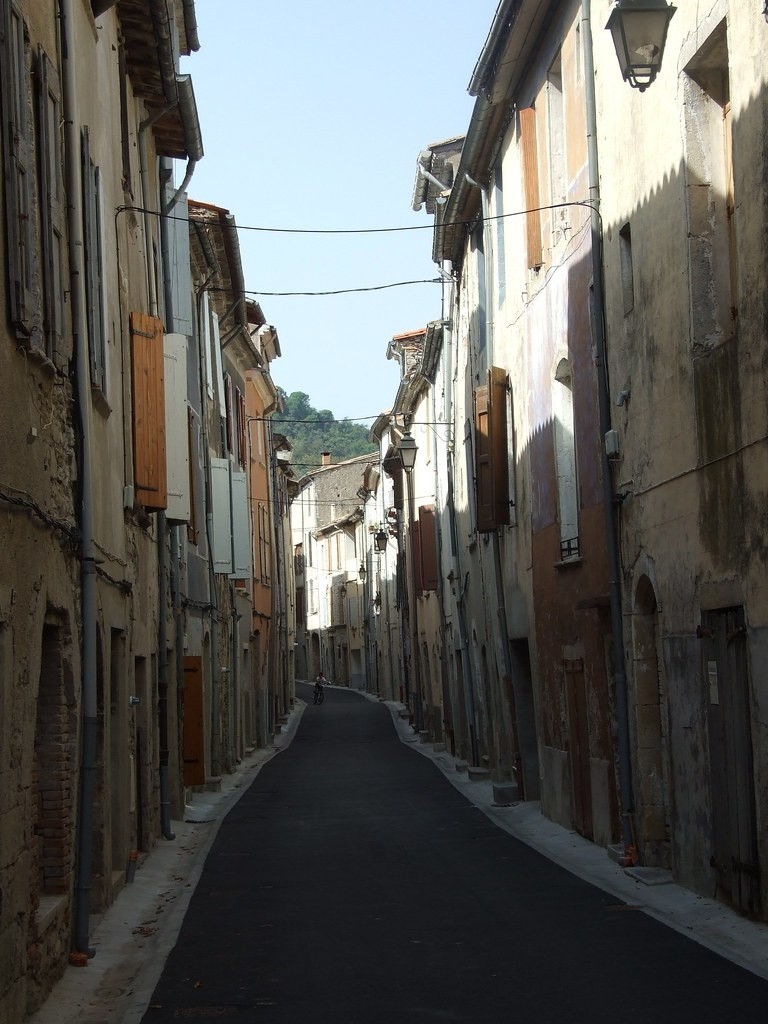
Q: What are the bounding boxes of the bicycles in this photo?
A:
[312,680,328,705]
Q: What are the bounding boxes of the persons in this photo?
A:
[312,672,326,703]
